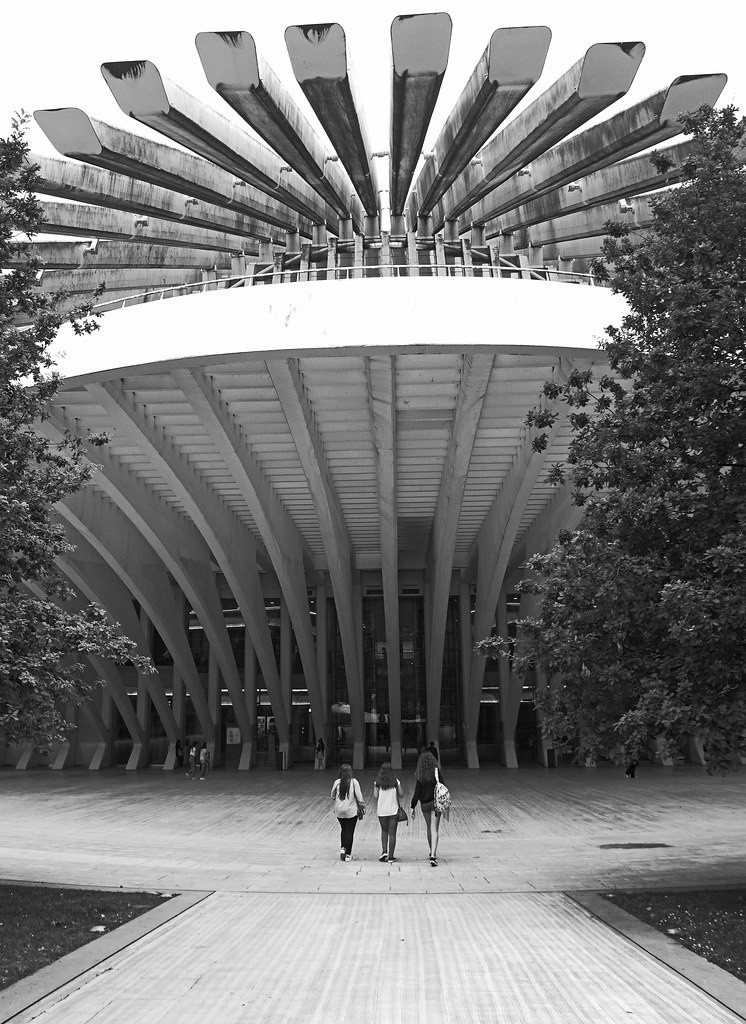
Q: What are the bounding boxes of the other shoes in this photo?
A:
[345,855,355,861]
[388,857,397,863]
[378,853,387,861]
[340,848,345,860]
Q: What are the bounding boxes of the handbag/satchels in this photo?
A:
[357,805,363,820]
[398,807,409,826]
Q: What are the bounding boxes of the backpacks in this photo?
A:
[433,766,452,812]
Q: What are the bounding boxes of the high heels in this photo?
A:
[429,857,438,867]
[429,853,437,862]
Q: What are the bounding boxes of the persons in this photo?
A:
[186,741,200,779]
[427,742,438,761]
[410,751,446,867]
[175,739,184,770]
[624,759,639,779]
[378,711,385,723]
[183,738,191,775]
[199,742,210,780]
[315,738,325,771]
[331,764,366,862]
[373,762,404,863]
